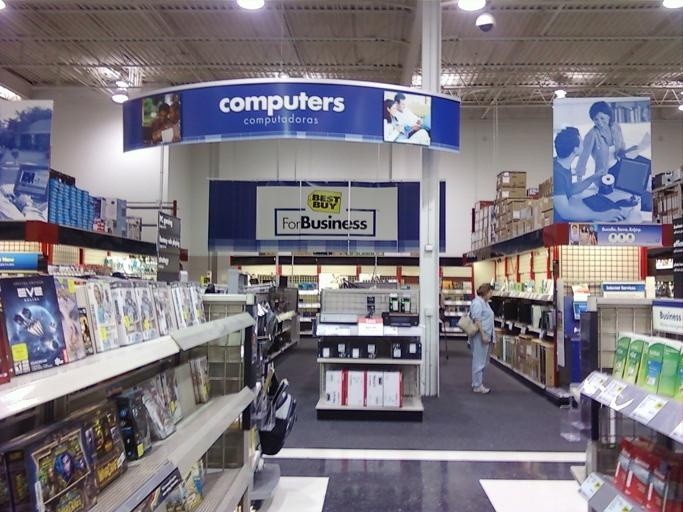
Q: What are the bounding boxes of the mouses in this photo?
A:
[611,216,626,222]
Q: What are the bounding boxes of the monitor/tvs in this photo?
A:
[615,158,651,207]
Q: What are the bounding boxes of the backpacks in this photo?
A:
[258,361,297,455]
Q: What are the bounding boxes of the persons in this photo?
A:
[150,102,181,145]
[470,283,495,394]
[384,93,431,144]
[553,101,626,222]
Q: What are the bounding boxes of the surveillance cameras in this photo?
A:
[475,13,495,32]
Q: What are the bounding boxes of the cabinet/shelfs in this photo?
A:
[570,296,683,512]
[469,222,664,406]
[315,280,427,420]
[2,218,324,512]
[438,256,473,338]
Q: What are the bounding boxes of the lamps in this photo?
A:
[112,81,129,104]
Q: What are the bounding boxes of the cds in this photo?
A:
[601,174,615,186]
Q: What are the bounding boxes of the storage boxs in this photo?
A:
[470,171,553,251]
[653,167,683,225]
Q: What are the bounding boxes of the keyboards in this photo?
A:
[582,193,621,213]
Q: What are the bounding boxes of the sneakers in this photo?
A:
[472,384,490,393]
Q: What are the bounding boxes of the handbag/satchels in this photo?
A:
[457,314,478,337]
